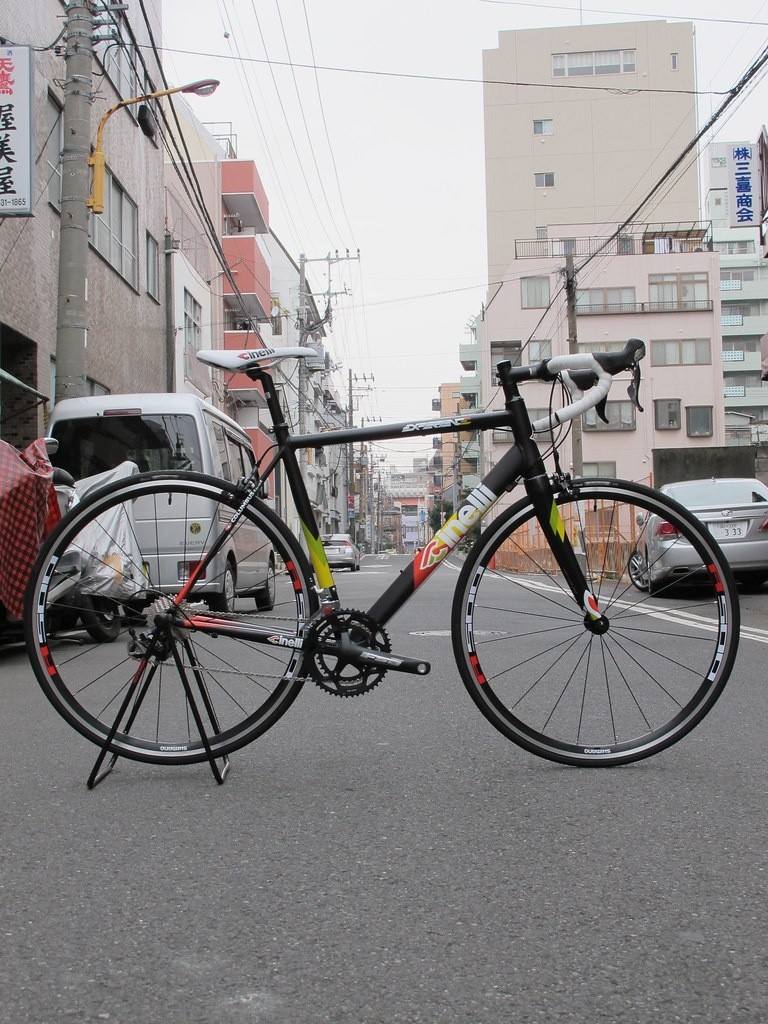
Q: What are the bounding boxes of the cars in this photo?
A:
[319,533,360,571]
[627,477,768,595]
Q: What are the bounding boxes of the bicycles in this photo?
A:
[20,343,741,789]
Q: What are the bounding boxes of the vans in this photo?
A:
[45,393,276,616]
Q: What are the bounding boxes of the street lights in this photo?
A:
[54,1,219,396]
